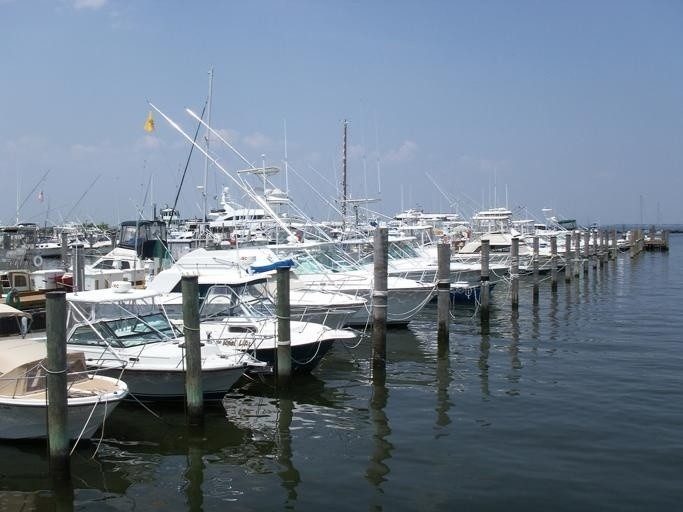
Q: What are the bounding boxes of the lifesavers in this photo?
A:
[437,230,448,241]
[6,288,21,308]
